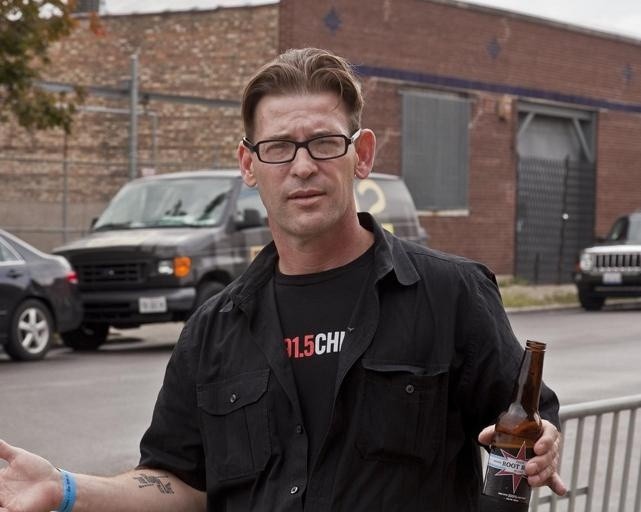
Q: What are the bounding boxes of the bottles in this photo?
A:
[478,339,547,512]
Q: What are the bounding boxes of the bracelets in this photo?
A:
[55,468,76,511]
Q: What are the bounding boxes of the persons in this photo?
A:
[0,47,567,512]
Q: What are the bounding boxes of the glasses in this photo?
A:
[241,128,369,164]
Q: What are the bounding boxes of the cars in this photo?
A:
[0,231,83,363]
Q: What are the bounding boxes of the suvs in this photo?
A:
[573,211,640,311]
[51,167,427,350]
